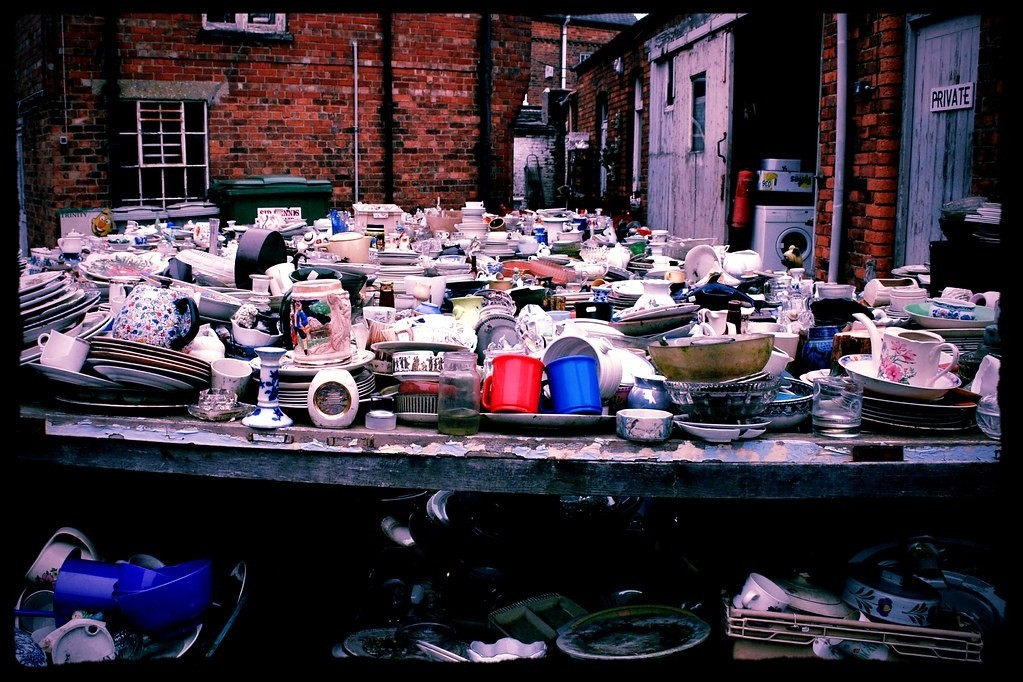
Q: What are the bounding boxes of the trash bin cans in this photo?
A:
[210,176,334,226]
[112,203,219,231]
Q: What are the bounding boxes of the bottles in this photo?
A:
[633,280,676,311]
[439,353,480,435]
[290,280,353,366]
[801,325,841,372]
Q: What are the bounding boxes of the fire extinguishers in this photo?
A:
[732,168,756,227]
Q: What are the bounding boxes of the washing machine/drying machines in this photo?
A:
[753,206,814,275]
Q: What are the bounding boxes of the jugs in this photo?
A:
[112,278,200,350]
[314,233,373,264]
[852,313,961,387]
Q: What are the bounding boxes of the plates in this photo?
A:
[334,590,710,661]
[18,206,1002,440]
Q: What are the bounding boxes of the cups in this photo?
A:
[539,355,603,415]
[738,572,789,612]
[481,354,545,415]
[37,327,90,373]
[811,375,864,437]
[210,358,254,401]
[19,527,117,665]
[830,609,896,660]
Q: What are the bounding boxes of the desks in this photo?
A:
[929,240,1003,299]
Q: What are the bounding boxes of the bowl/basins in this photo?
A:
[394,350,446,372]
[391,392,440,412]
[230,317,285,348]
[112,558,213,625]
[616,408,674,441]
[846,565,941,626]
[662,374,792,422]
[647,332,776,383]
